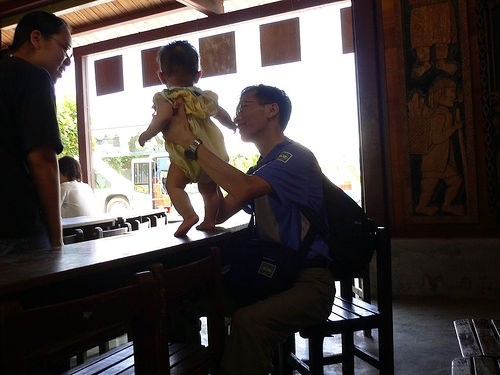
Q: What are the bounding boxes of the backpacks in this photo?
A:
[289,175,374,281]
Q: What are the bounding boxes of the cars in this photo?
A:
[73,154,149,220]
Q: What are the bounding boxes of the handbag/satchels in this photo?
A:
[218,228,302,306]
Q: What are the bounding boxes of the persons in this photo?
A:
[0,10,72,248]
[138,39,240,238]
[152,83,336,375]
[58,155,99,218]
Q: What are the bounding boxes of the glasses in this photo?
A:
[42,32,72,59]
[236,101,272,115]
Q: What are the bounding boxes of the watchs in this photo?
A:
[184,137,204,162]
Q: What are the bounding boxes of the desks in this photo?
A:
[62,209,167,244]
[0,222,249,375]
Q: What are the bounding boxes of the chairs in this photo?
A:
[449,319,500,375]
[0,213,392,375]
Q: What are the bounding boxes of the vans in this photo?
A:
[130,150,176,215]
